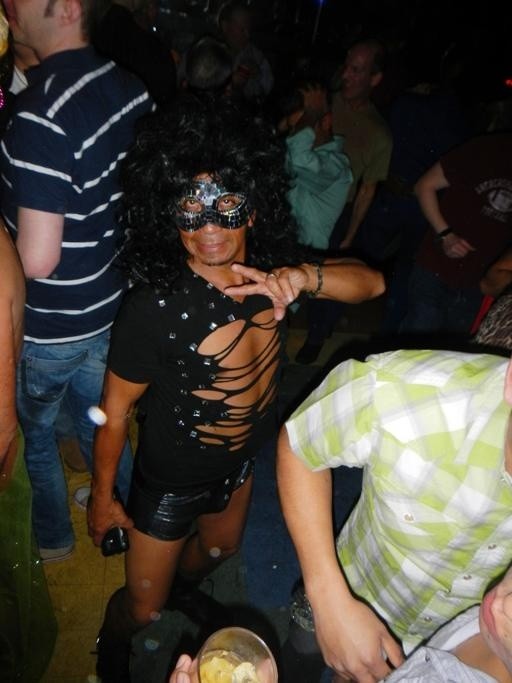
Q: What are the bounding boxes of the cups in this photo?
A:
[188,624,278,682]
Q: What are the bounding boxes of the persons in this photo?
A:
[1,217,60,680]
[163,563,509,680]
[277,347,511,681]
[83,103,390,682]
[1,1,511,346]
[0,0,159,564]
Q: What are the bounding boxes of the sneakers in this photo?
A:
[36,546,75,562]
[73,487,92,511]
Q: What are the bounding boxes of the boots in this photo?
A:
[97,585,161,682]
